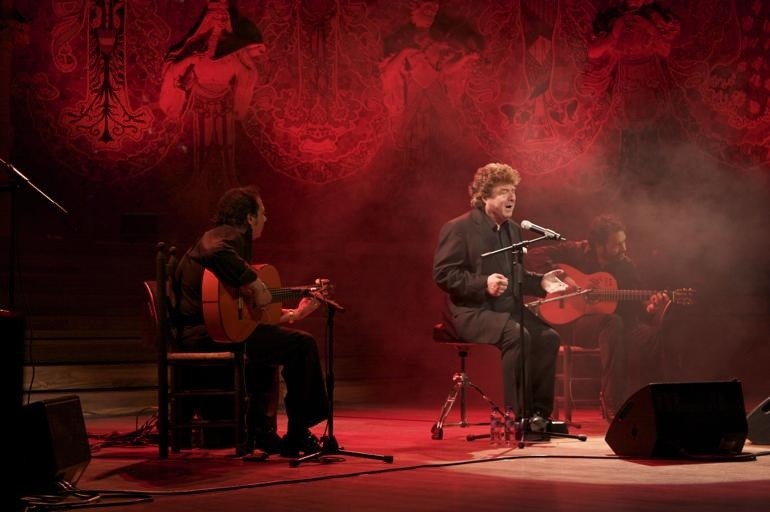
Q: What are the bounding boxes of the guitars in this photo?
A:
[201,263,335,345]
[538,263,697,326]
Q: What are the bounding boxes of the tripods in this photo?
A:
[291,320,394,464]
[468,281,587,445]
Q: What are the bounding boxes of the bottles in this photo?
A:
[191,414,205,450]
[489,407,502,443]
[505,406,517,444]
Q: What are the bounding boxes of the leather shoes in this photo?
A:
[515,417,555,442]
[245,430,325,457]
[597,388,619,425]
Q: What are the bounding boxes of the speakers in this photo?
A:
[747,395,770,444]
[605,380,749,457]
[27,395,91,473]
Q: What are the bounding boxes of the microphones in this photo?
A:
[521,220,564,241]
[303,288,345,314]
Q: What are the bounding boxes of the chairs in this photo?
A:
[137,274,279,459]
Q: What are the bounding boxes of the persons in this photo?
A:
[526,214,670,421]
[171,187,344,458]
[431,162,570,441]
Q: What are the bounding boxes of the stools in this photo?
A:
[423,320,510,442]
[549,342,603,424]
